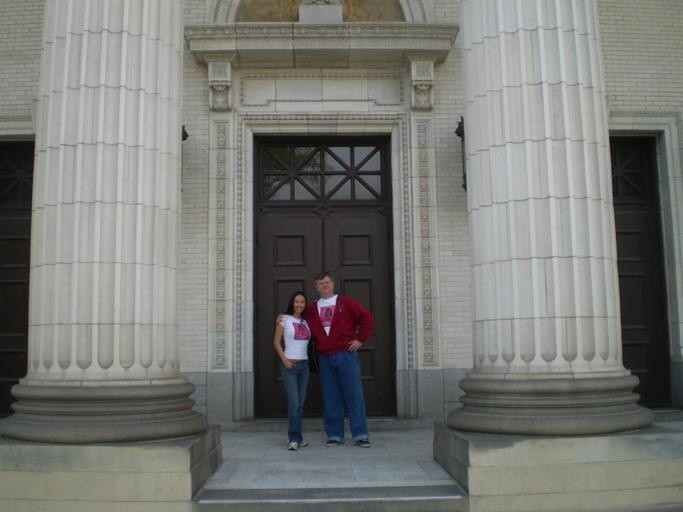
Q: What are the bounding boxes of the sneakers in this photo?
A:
[326,439,342,447]
[355,439,371,447]
[287,440,308,450]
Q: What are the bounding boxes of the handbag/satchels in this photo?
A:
[307,340,319,373]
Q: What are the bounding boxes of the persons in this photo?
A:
[275,270,374,448]
[273,291,312,451]
